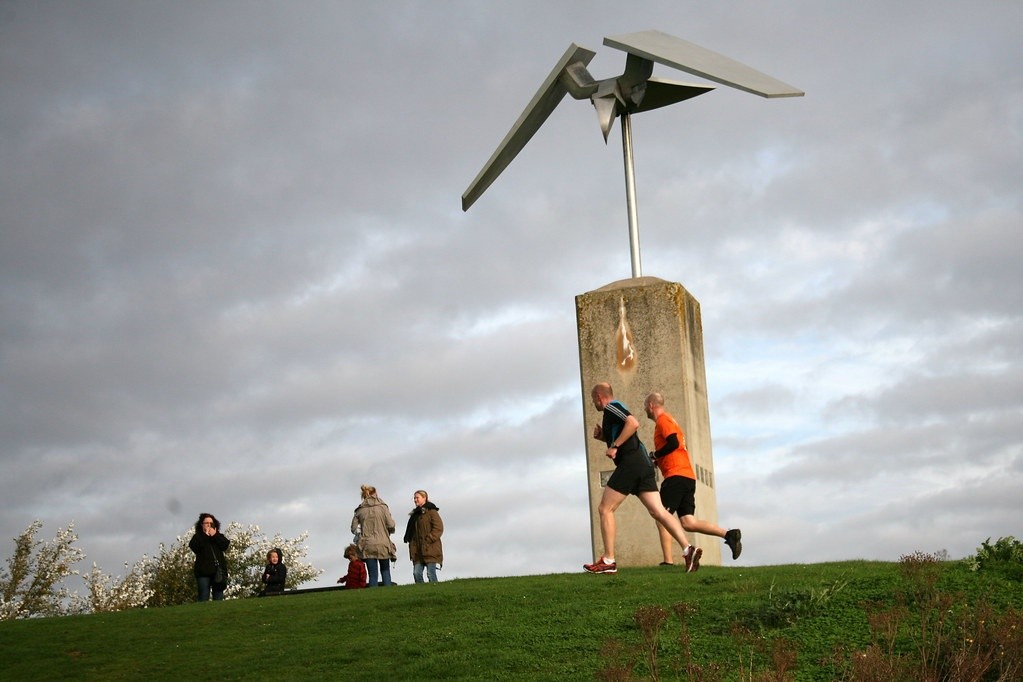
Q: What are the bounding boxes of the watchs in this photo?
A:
[650,452,656,458]
[611,442,618,448]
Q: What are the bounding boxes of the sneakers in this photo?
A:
[683,546,702,572]
[583,557,618,574]
[725,529,742,560]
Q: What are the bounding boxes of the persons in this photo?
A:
[337,544,367,591]
[583,383,702,576]
[644,392,742,565]
[258,548,286,595]
[404,490,444,584]
[188,513,229,602]
[351,485,395,586]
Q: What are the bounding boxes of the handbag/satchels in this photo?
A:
[213,567,227,590]
